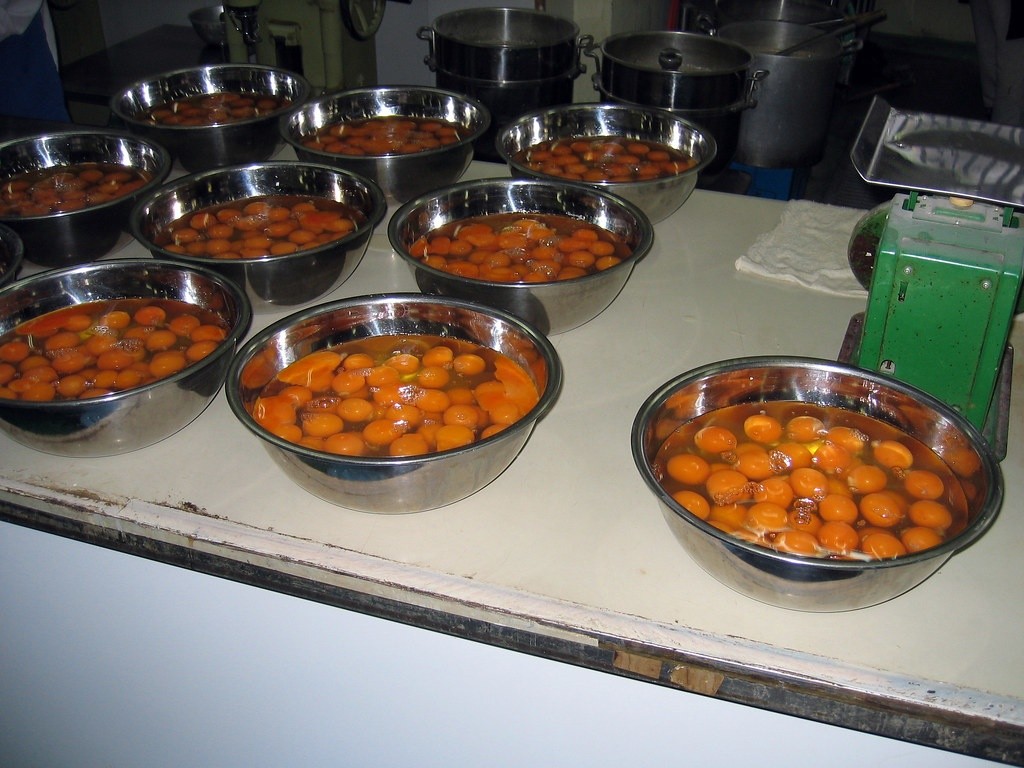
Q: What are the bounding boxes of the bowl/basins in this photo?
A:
[387,176,654,337]
[187,5,228,49]
[110,63,310,174]
[225,292,564,514]
[0,225,24,290]
[495,104,717,226]
[631,355,1004,612]
[131,160,387,317]
[0,129,172,267]
[0,258,253,457]
[281,86,491,204]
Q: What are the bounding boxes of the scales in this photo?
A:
[836,95,1024,463]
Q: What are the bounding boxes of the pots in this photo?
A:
[680,0,863,87]
[417,6,593,164]
[583,30,769,174]
[694,13,863,167]
